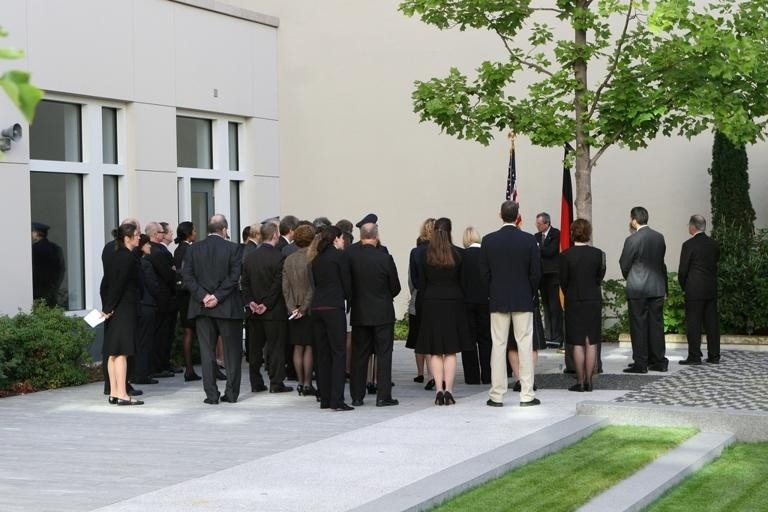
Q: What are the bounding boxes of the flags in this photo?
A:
[505,138,523,231]
[559,144,574,314]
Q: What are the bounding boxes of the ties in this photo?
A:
[541,233,546,247]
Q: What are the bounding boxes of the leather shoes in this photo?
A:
[442,380,446,390]
[172,366,184,373]
[127,389,142,396]
[586,383,594,392]
[221,394,237,403]
[116,395,144,405]
[487,399,505,407]
[563,367,578,375]
[414,375,424,384]
[108,395,117,403]
[707,357,720,365]
[331,401,355,411]
[532,384,536,390]
[425,379,436,391]
[678,357,703,366]
[321,401,331,409]
[627,363,637,367]
[377,397,399,407]
[568,383,585,392]
[622,364,648,374]
[352,399,364,407]
[215,368,226,380]
[204,397,219,405]
[649,359,670,373]
[251,384,268,393]
[129,376,159,385]
[184,372,202,381]
[150,369,175,378]
[270,385,293,393]
[513,381,522,392]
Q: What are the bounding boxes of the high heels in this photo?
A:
[296,384,303,396]
[444,391,456,406]
[304,385,318,396]
[373,383,377,395]
[434,391,444,406]
[314,391,323,402]
[366,382,372,394]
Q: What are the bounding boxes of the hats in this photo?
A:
[30,220,51,231]
[259,215,280,226]
[355,213,378,227]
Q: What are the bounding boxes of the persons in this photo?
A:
[160,222,184,374]
[275,215,299,381]
[627,221,637,367]
[130,234,159,386]
[182,214,245,404]
[622,206,669,373]
[405,236,426,382]
[216,335,225,370]
[559,219,605,393]
[344,223,398,407]
[348,214,395,394]
[480,201,541,407]
[241,220,293,393]
[243,226,250,243]
[242,223,264,364]
[282,221,316,256]
[414,216,464,407]
[410,218,447,390]
[678,215,721,365]
[145,221,175,377]
[282,224,317,396]
[336,219,353,384]
[309,226,355,410]
[313,218,328,233]
[263,218,280,237]
[174,221,227,382]
[507,285,543,392]
[461,226,492,385]
[533,212,563,349]
[31,222,66,310]
[307,230,320,401]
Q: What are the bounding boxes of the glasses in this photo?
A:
[535,222,546,227]
[157,231,166,234]
